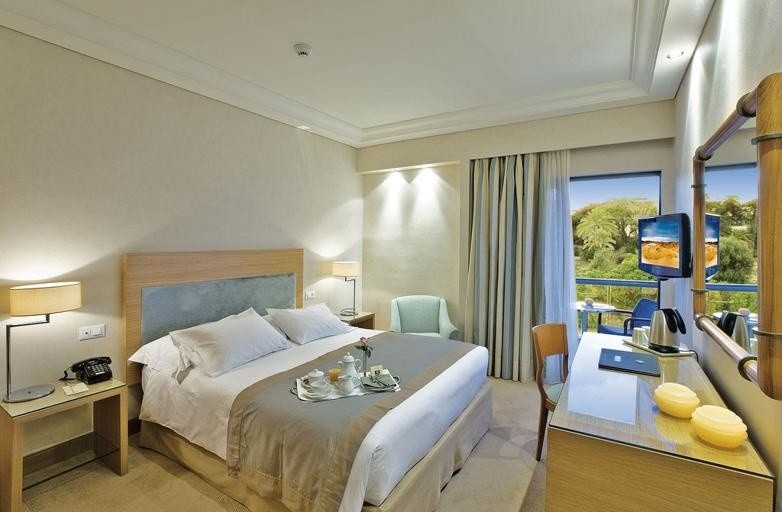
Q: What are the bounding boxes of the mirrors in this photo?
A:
[690,73,782,398]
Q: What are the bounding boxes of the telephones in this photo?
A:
[71,357,112,384]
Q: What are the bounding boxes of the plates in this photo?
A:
[303,390,328,400]
[302,374,330,388]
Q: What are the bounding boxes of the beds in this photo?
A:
[116,248,492,512]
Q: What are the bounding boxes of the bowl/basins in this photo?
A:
[585,297,594,305]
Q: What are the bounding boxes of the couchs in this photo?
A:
[387,293,458,343]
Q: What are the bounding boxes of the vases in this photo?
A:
[362,351,367,378]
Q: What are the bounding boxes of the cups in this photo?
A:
[328,368,341,383]
[632,327,650,347]
[307,368,325,383]
[750,338,759,354]
[309,381,328,395]
[641,325,650,337]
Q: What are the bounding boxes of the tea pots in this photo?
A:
[337,375,362,396]
[336,352,362,387]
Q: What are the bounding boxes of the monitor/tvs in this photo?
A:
[704,213,721,282]
[637,212,691,278]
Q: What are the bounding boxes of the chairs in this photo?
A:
[529,321,574,462]
[596,296,657,337]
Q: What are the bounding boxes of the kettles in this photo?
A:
[649,307,688,356]
[716,312,750,353]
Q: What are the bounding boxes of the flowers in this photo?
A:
[354,337,374,378]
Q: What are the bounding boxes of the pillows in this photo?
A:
[166,308,293,376]
[126,328,194,384]
[264,301,357,345]
[260,312,289,341]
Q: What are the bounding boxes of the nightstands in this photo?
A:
[339,312,378,332]
[0,378,132,512]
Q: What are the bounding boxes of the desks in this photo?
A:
[539,328,782,512]
[571,298,614,334]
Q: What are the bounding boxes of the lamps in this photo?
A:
[0,281,82,404]
[325,260,366,316]
[292,42,312,60]
